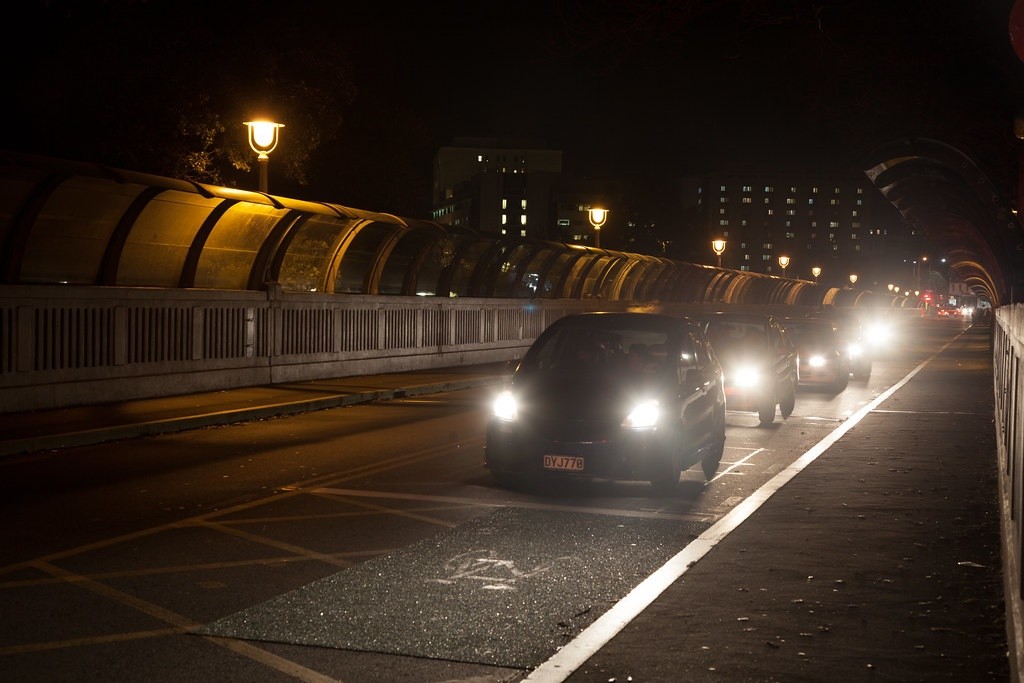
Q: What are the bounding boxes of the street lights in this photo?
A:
[851,274,920,299]
[242,114,285,193]
[587,203,610,249]
[779,254,790,278]
[712,237,727,267]
[812,265,822,283]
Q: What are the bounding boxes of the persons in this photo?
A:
[573,340,596,371]
[627,344,656,375]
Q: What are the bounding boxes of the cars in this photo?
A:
[778,316,847,390]
[695,313,799,423]
[482,310,726,492]
[806,303,985,382]
